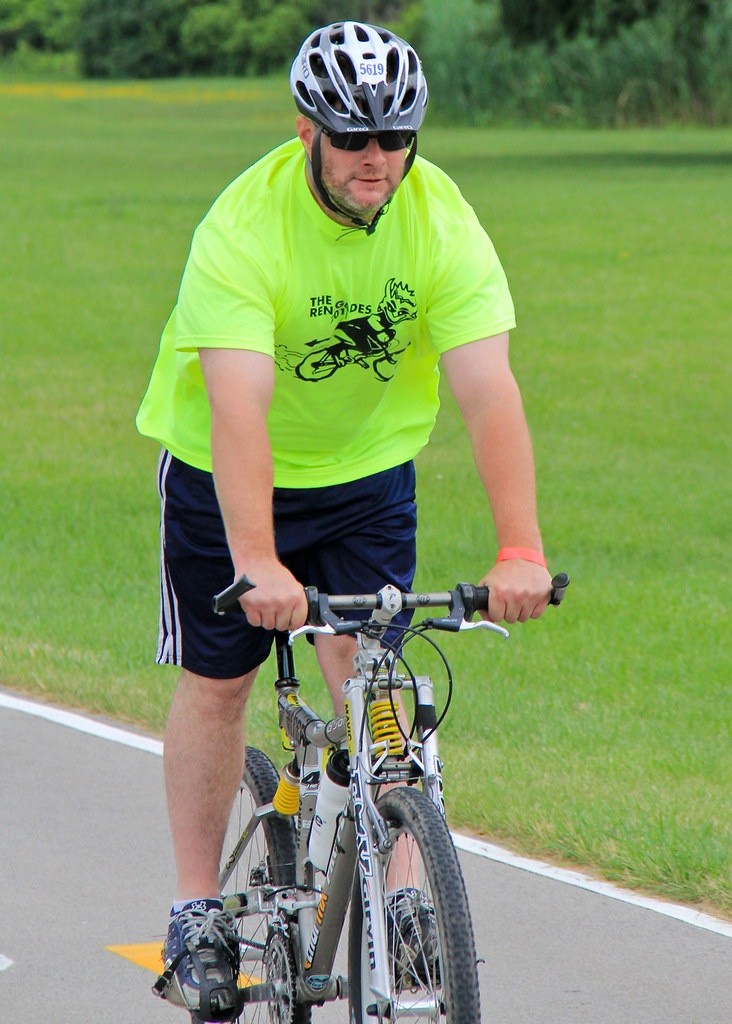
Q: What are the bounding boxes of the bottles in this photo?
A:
[308,749,353,871]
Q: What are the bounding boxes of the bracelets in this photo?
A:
[497,547,546,568]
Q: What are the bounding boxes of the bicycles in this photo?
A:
[189,567,571,1024]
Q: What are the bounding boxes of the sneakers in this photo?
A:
[161,899,240,1011]
[386,887,442,992]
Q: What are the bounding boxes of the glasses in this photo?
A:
[309,119,413,151]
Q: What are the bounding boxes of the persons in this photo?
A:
[134,23,551,1019]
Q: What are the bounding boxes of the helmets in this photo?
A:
[290,21,430,133]
